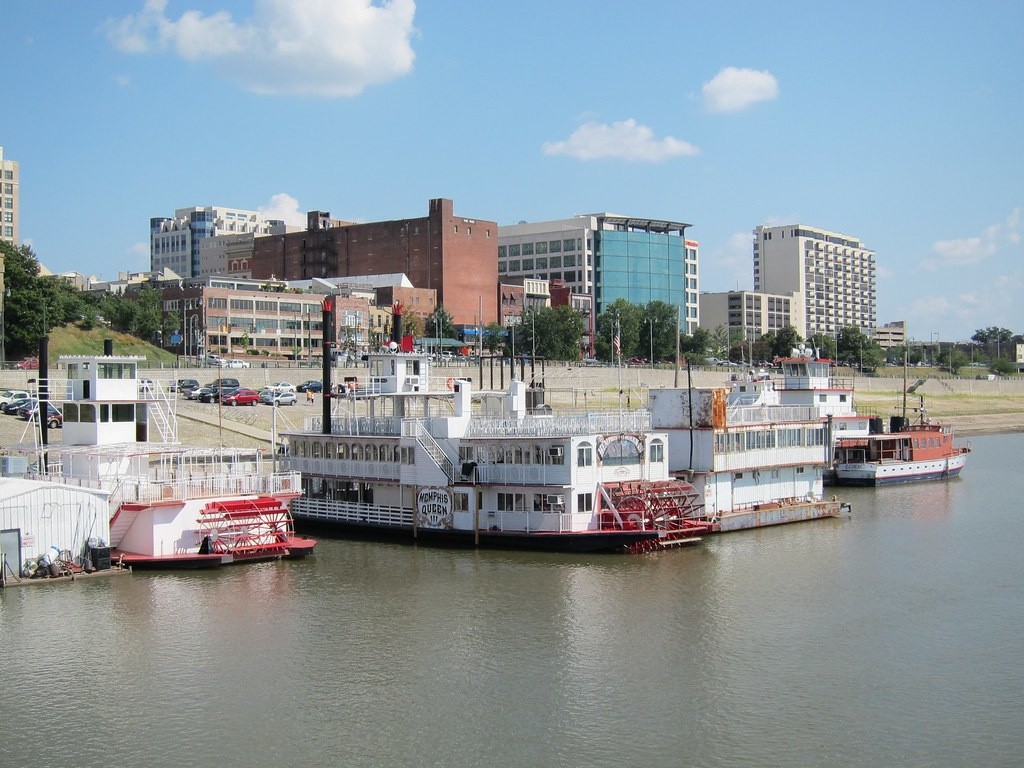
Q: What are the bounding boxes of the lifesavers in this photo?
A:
[447,378,455,389]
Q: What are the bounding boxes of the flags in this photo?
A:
[614,328,622,357]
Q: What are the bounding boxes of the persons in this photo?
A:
[305,381,336,406]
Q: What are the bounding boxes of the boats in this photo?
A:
[1,352,971,590]
